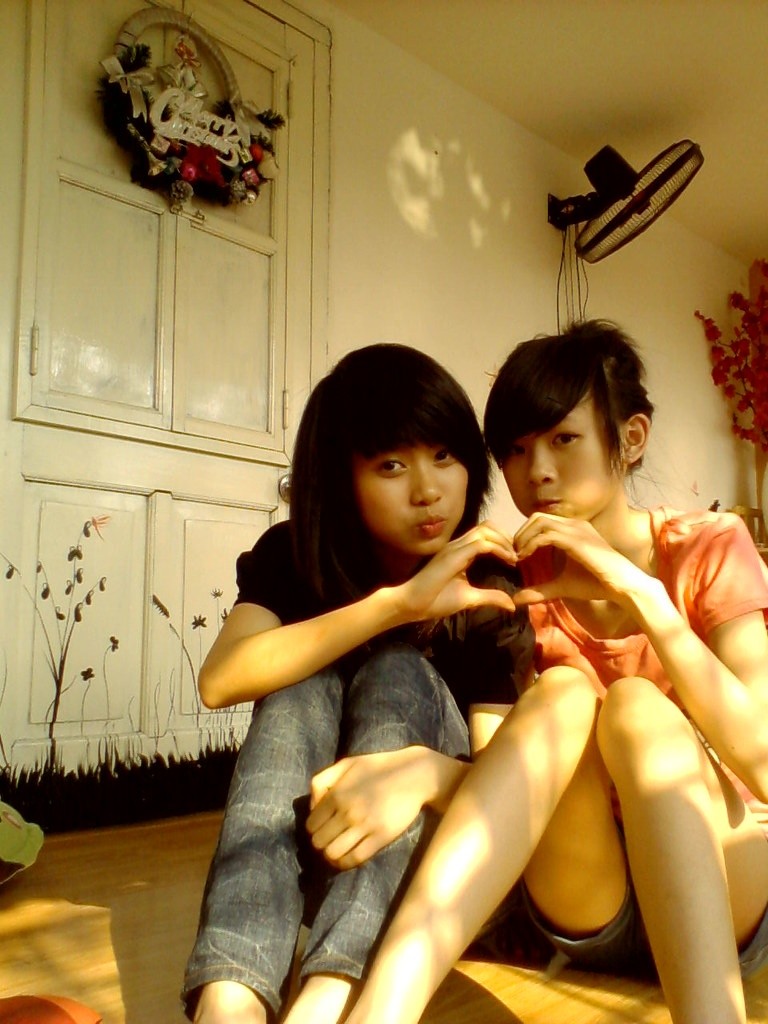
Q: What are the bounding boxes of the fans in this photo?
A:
[546,138,705,265]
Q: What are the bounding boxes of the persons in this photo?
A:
[181,342,536,1024]
[349,319,768,1023]
